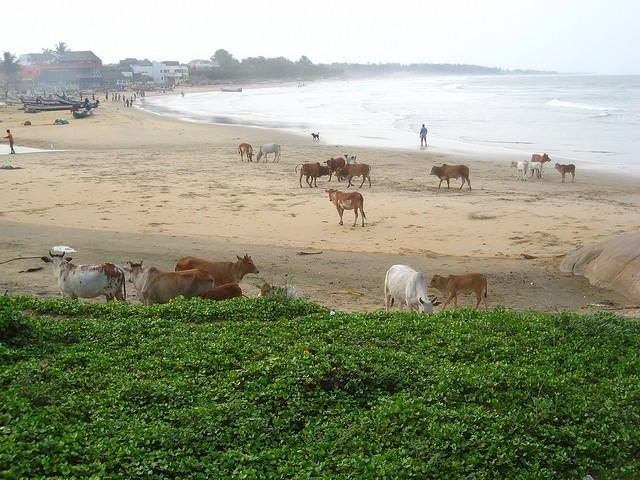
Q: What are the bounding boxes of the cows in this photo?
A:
[256,143,281,164]
[335,163,372,188]
[429,164,472,191]
[324,157,346,182]
[202,284,242,301]
[510,160,530,181]
[325,189,368,227]
[255,283,297,300]
[531,162,543,179]
[294,161,333,188]
[123,258,215,307]
[383,264,441,317]
[174,253,259,288]
[430,272,489,312]
[531,153,552,178]
[555,162,576,183]
[41,249,126,304]
[237,142,254,163]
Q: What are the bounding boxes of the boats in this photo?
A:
[58,99,99,108]
[20,98,40,104]
[73,110,90,119]
[221,87,242,93]
[38,95,58,102]
[56,93,100,104]
[24,105,79,111]
[38,97,59,105]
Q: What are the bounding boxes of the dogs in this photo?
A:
[344,154,358,165]
[311,133,320,141]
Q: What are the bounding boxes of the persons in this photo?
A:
[419,122,431,149]
[0,77,186,108]
[2,129,15,155]
